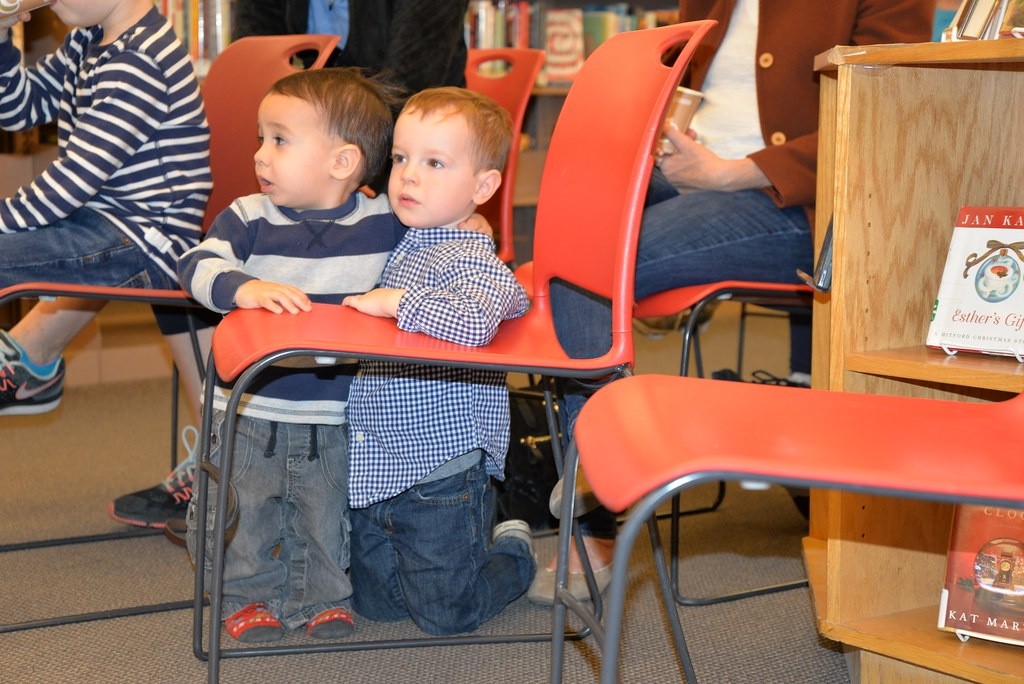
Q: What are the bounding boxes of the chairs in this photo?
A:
[191,16,718,684]
[511,256,814,606]
[465,49,548,270]
[0,34,342,481]
[563,371,1024,684]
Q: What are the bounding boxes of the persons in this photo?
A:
[0,0,475,533]
[339,85,537,638]
[0,0,212,309]
[523,0,937,608]
[176,66,494,643]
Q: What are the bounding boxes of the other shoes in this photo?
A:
[550,466,602,520]
[712,367,811,388]
[530,558,616,606]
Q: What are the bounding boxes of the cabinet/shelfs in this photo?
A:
[513,84,567,210]
[802,36,1024,684]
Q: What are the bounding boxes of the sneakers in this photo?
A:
[108,425,199,528]
[0,329,66,417]
[492,519,538,563]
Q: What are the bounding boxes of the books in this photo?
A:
[154,0,218,67]
[940,0,1024,42]
[0,125,41,155]
[467,0,682,90]
[936,501,1024,648]
[925,204,1023,357]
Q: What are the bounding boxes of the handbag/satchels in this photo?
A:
[491,375,626,531]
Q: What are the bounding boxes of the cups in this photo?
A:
[0,0,52,22]
[656,86,703,154]
